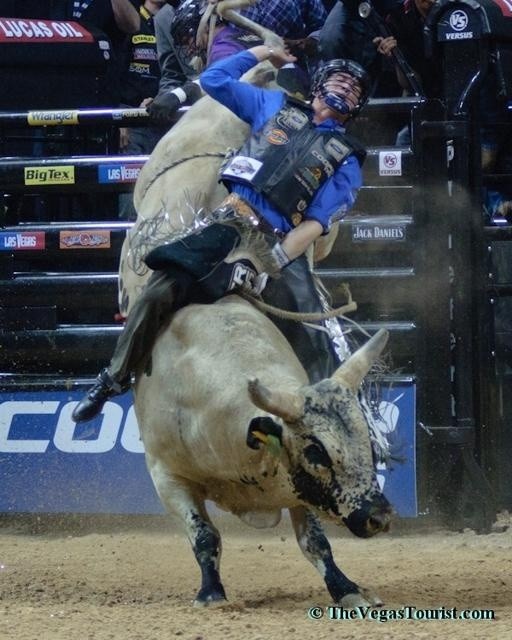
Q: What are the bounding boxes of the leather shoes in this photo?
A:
[71,372,131,423]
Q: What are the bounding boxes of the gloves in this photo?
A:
[240,239,296,298]
[145,93,181,123]
[282,38,319,60]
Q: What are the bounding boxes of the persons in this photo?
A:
[68,40,370,425]
[58,1,511,246]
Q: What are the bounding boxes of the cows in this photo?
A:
[112,1,397,614]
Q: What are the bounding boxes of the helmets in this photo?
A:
[170,1,221,70]
[309,59,372,121]
